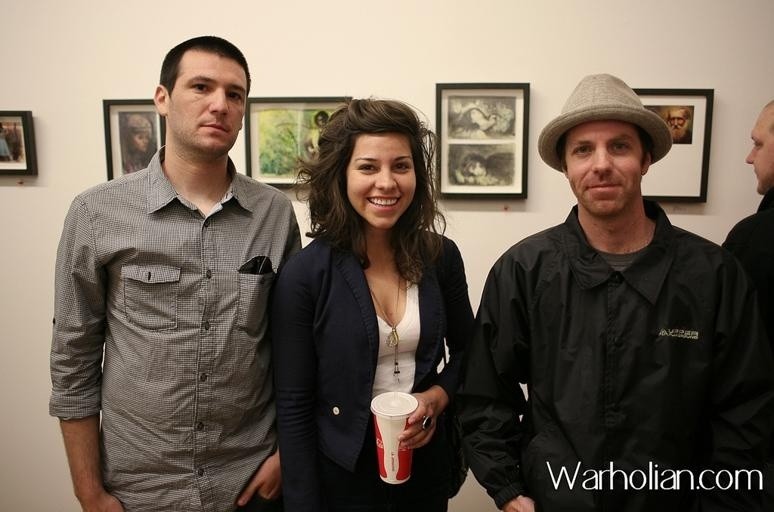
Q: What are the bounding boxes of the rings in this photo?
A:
[421,415,432,430]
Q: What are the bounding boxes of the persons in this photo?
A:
[665,107,693,145]
[49,36,306,511]
[121,115,154,175]
[272,99,476,512]
[0,122,21,162]
[451,105,515,139]
[455,74,769,512]
[458,152,514,185]
[717,101,773,332]
[304,111,329,159]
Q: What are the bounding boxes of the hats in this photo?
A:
[538,75,672,171]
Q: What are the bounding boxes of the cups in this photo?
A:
[370,392,419,485]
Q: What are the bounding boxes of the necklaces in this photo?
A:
[370,274,402,384]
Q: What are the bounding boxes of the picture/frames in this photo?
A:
[631,88,715,203]
[245,96,351,190]
[103,100,166,184]
[435,81,529,200]
[0,110,38,178]
